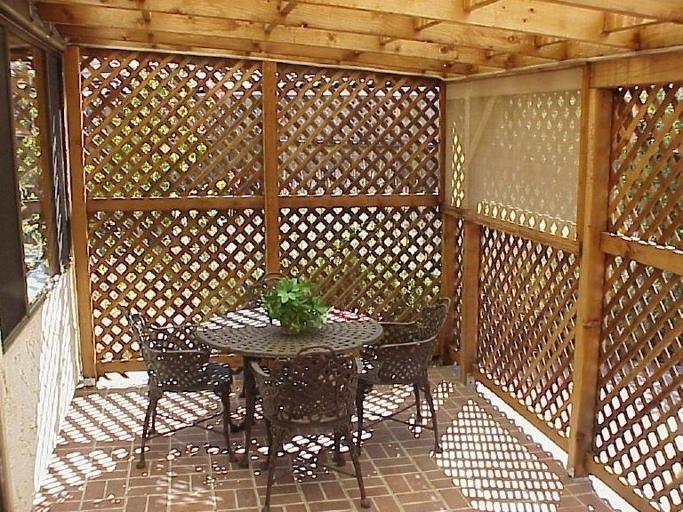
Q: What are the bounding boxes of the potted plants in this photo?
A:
[263,276,328,336]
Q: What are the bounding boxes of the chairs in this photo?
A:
[354,294,452,454]
[248,348,373,508]
[250,272,283,306]
[125,313,242,468]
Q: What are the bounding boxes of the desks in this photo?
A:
[197,307,383,467]
